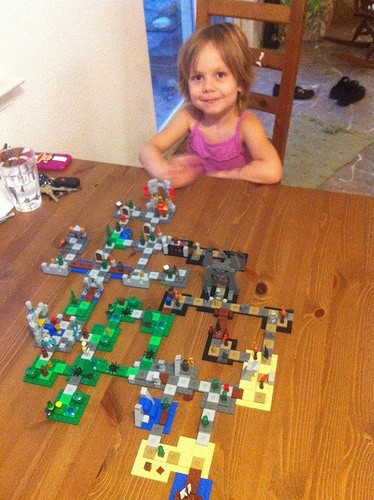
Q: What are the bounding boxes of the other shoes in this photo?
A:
[272,83,316,99]
[330,80,359,98]
[338,86,365,106]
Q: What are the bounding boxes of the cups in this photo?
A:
[0,147,43,213]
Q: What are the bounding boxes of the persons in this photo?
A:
[138,21,284,192]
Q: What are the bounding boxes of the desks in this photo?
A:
[1,147,374,499]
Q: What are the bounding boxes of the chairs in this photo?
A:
[196,0,308,164]
